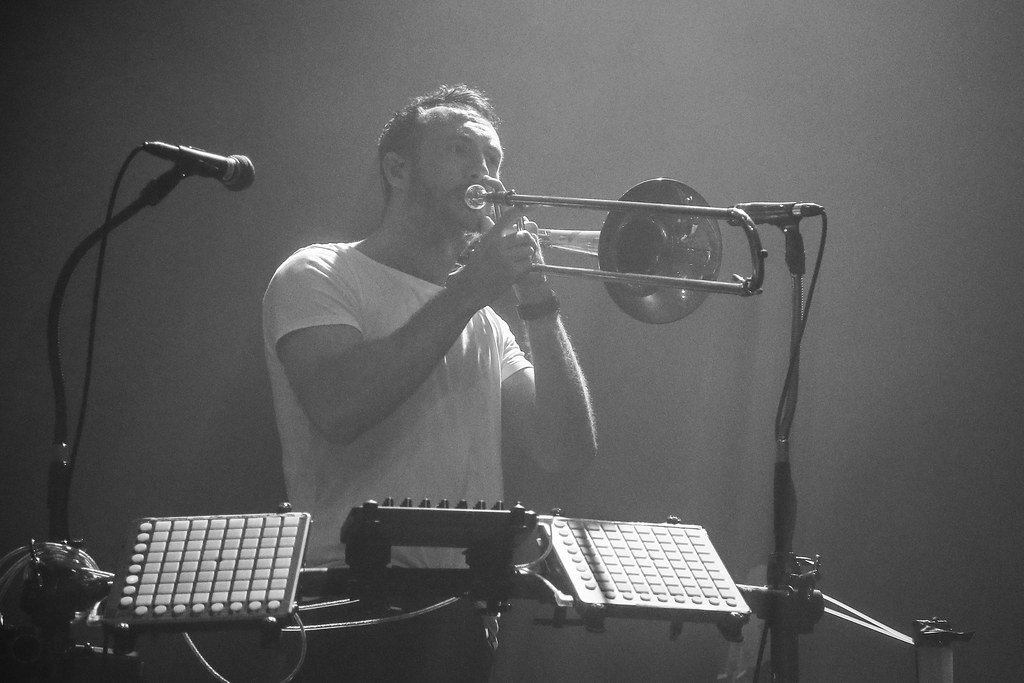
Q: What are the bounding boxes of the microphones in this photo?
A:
[727,202,824,226]
[143,141,255,192]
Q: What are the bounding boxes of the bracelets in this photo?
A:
[516,297,562,320]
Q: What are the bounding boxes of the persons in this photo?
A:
[260,87,598,683]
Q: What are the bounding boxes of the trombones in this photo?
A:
[459,178,768,328]
